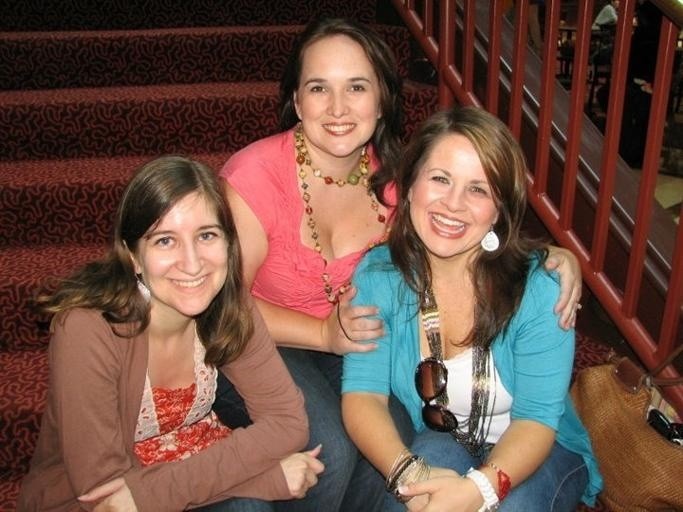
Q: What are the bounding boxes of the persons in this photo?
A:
[337,105,605,512]
[202,13,585,511]
[13,154,328,509]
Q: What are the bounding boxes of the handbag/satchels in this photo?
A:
[571,358,683,512]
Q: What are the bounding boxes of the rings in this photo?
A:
[572,298,582,310]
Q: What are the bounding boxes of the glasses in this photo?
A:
[415,358,458,432]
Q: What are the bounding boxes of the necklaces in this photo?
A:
[295,119,389,189]
[417,274,497,465]
[286,125,391,309]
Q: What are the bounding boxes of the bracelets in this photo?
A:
[477,460,511,503]
[382,447,432,504]
[461,466,499,512]
